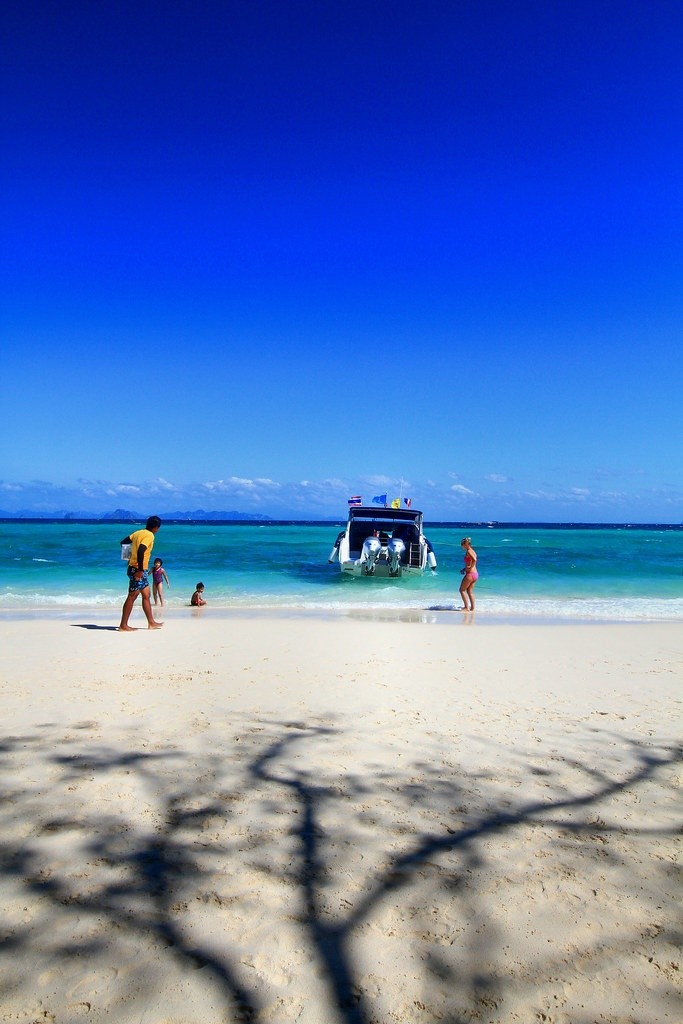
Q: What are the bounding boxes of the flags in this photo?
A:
[372,495,386,504]
[391,499,400,509]
[404,498,411,508]
[348,496,362,507]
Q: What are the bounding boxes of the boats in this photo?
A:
[488,521,499,525]
[330,507,438,581]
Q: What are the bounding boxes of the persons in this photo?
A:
[459,538,478,611]
[120,516,164,631]
[148,558,170,606]
[191,582,206,607]
